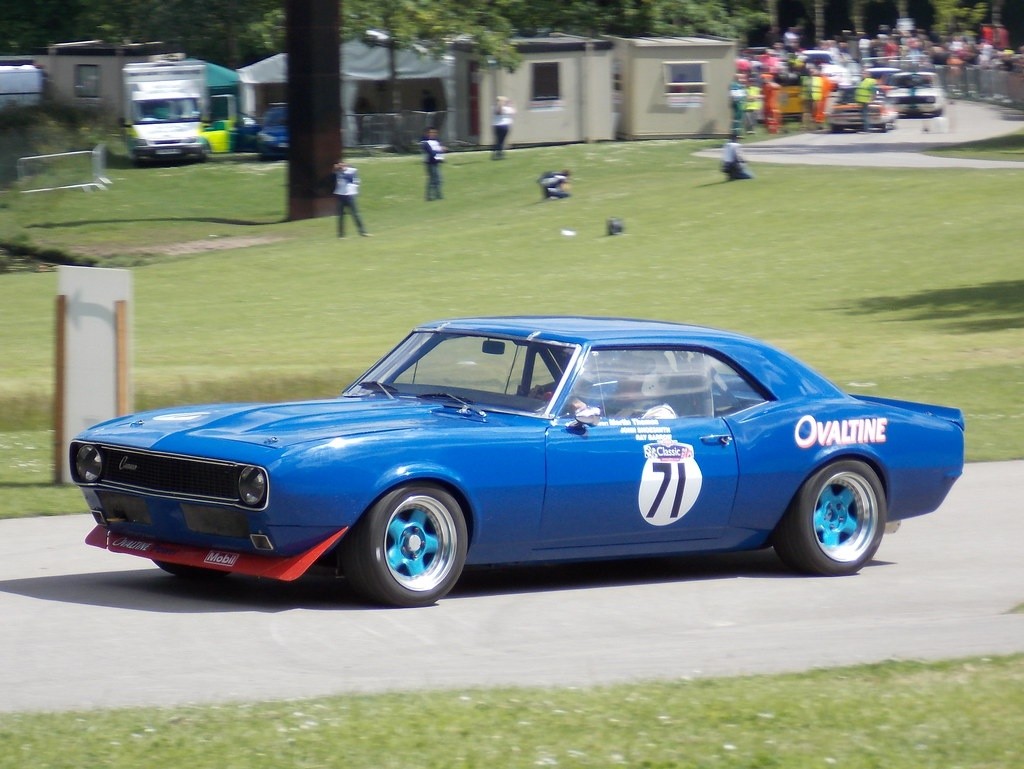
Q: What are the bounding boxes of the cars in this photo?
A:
[253,102,290,160]
[68,316,966,609]
[859,56,899,84]
[886,71,946,119]
[800,51,863,90]
[825,87,900,133]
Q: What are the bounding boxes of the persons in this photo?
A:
[605,349,680,420]
[728,18,1024,139]
[723,134,752,180]
[541,168,572,198]
[489,96,513,159]
[421,127,445,201]
[330,161,371,239]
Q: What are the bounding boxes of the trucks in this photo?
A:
[119,57,211,164]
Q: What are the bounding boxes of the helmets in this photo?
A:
[630,349,679,398]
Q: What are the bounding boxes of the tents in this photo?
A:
[189,57,241,121]
[237,30,457,152]
